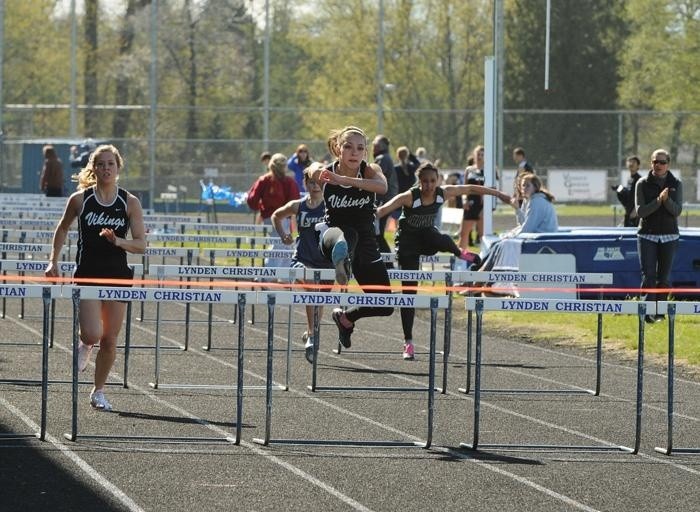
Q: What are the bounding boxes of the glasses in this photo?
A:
[651,159,668,164]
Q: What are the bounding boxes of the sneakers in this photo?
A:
[332,241,352,285]
[76,343,94,372]
[331,308,355,348]
[90,389,106,410]
[304,338,313,363]
[403,342,414,359]
[459,248,481,264]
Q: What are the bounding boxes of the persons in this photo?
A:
[45,145,148,411]
[39,145,63,197]
[433,159,443,228]
[371,136,398,270]
[454,171,536,289]
[634,149,682,323]
[69,138,100,193]
[271,162,330,363]
[286,144,318,198]
[465,157,485,247]
[458,145,484,250]
[460,175,558,297]
[413,146,435,186]
[393,147,420,193]
[375,161,517,360]
[611,156,642,227]
[512,147,536,209]
[308,126,394,348]
[247,154,300,283]
[259,152,273,171]
[443,173,462,208]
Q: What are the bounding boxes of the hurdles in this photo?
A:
[1,284,61,441]
[0,229,14,319]
[15,230,129,319]
[63,284,257,446]
[177,222,273,236]
[135,233,246,325]
[332,252,456,357]
[246,236,349,326]
[458,270,613,396]
[1,259,145,388]
[1,243,67,348]
[0,192,207,222]
[202,248,305,352]
[252,291,447,450]
[70,245,199,351]
[306,269,461,395]
[459,296,658,455]
[654,300,700,455]
[0,219,174,233]
[149,265,304,392]
